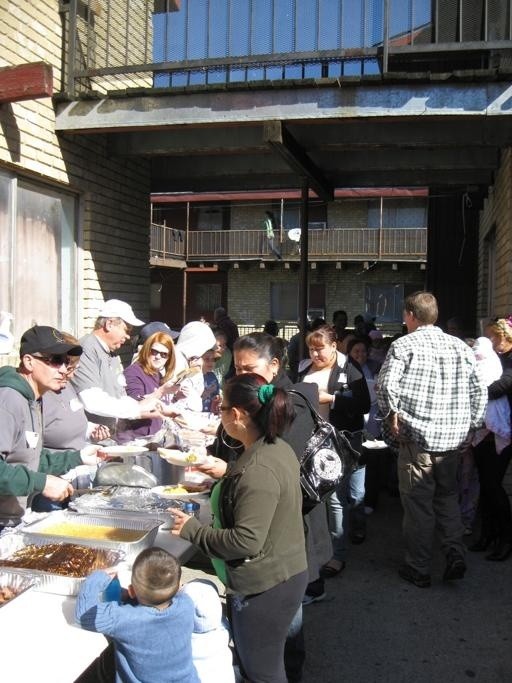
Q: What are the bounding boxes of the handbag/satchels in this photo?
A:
[296,390,363,516]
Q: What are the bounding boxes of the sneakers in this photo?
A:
[302,580,327,607]
[398,564,433,589]
[442,551,467,583]
[320,563,345,579]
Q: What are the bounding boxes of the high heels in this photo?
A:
[486,541,512,562]
[467,531,497,552]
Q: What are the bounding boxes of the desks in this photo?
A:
[0,495,215,683]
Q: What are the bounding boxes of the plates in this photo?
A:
[91,443,152,461]
[160,447,210,468]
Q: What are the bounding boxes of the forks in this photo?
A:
[66,485,104,495]
[169,369,191,387]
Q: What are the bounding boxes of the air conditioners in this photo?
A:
[307,308,325,322]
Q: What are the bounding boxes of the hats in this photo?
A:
[19,325,83,360]
[97,298,146,328]
[140,321,181,342]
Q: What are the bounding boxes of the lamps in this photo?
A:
[197,261,426,271]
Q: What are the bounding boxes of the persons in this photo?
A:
[1,291,512,683]
[259,211,282,259]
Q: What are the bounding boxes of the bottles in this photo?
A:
[185,500,200,522]
